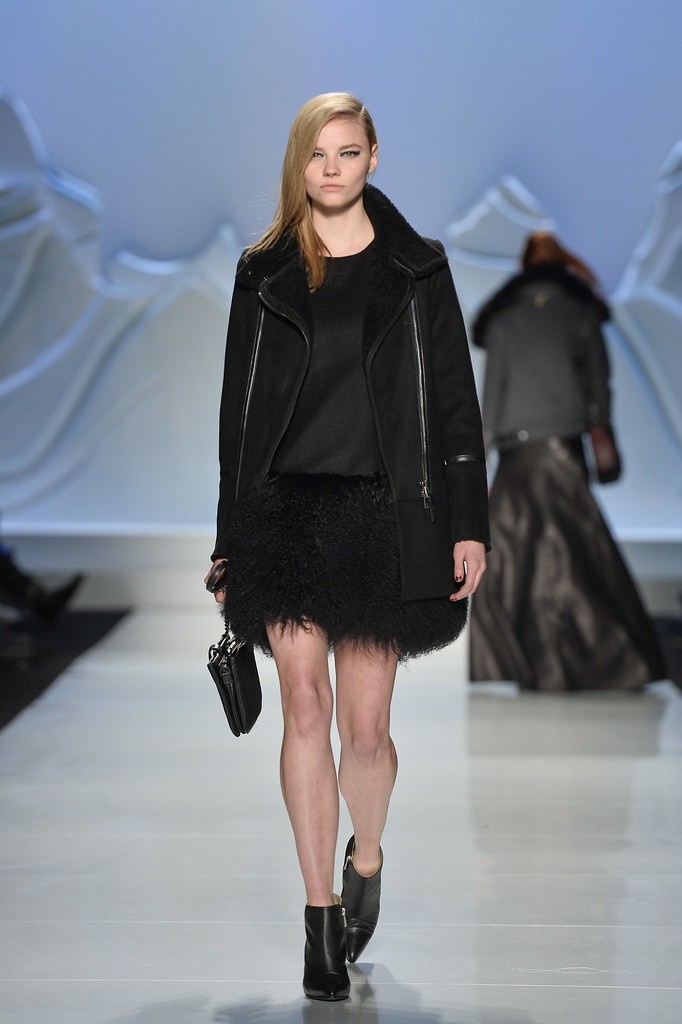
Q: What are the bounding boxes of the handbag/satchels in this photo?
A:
[207,634,262,737]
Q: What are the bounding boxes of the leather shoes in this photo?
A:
[303,904,351,1000]
[341,835,383,963]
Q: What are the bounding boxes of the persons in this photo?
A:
[203,91,493,1002]
[466,230,682,700]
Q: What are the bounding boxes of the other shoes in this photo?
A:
[29,575,82,623]
